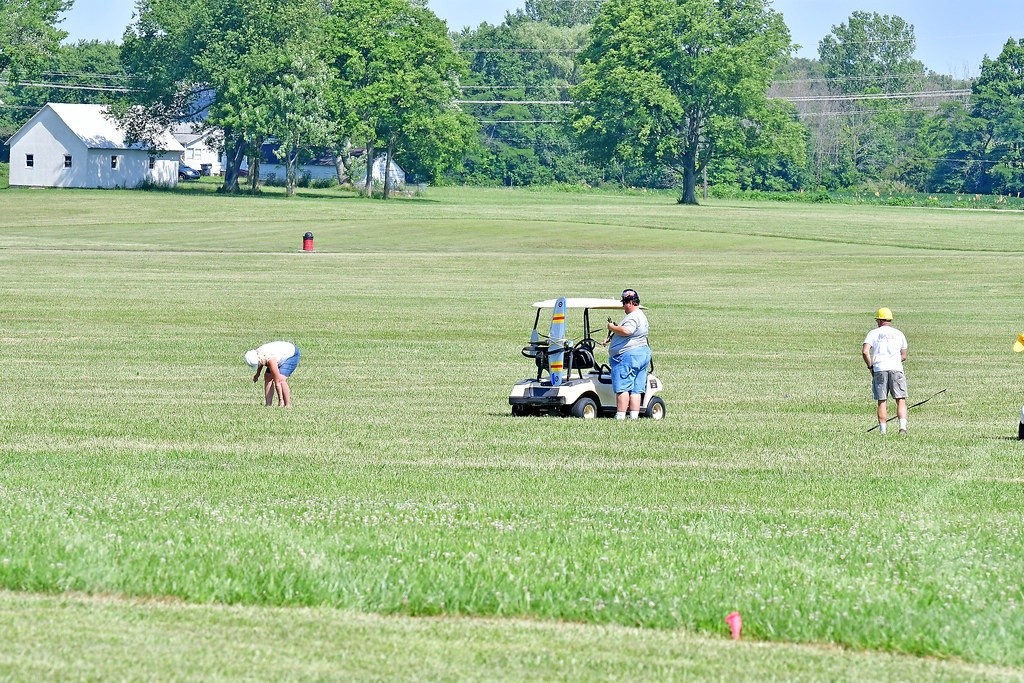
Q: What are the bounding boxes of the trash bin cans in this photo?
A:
[199,163,212,176]
[303,232,314,250]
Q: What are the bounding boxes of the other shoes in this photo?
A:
[899,429,906,434]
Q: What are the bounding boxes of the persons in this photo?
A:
[608,289,651,420]
[244,341,301,407]
[862,308,908,436]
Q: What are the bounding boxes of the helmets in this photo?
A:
[620,289,639,301]
[874,308,893,319]
[245,350,258,371]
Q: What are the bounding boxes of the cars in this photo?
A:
[179,159,202,182]
